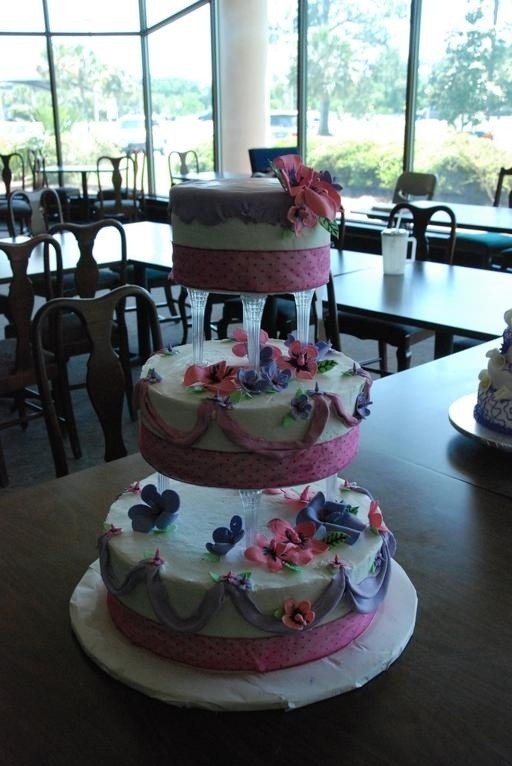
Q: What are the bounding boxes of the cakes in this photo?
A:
[473,309,512,436]
[137,336,371,490]
[168,177,332,294]
[98,472,398,675]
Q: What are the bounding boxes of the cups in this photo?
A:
[382,228,419,275]
